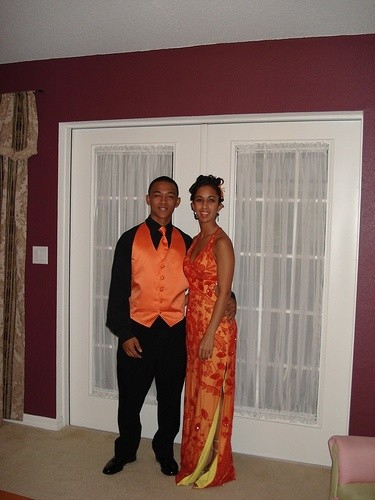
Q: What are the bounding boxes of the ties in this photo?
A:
[157,226,168,256]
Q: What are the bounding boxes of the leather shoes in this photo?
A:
[155,455,178,476]
[103,453,136,475]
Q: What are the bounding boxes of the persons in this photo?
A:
[102,176,236,476]
[175,174,237,490]
